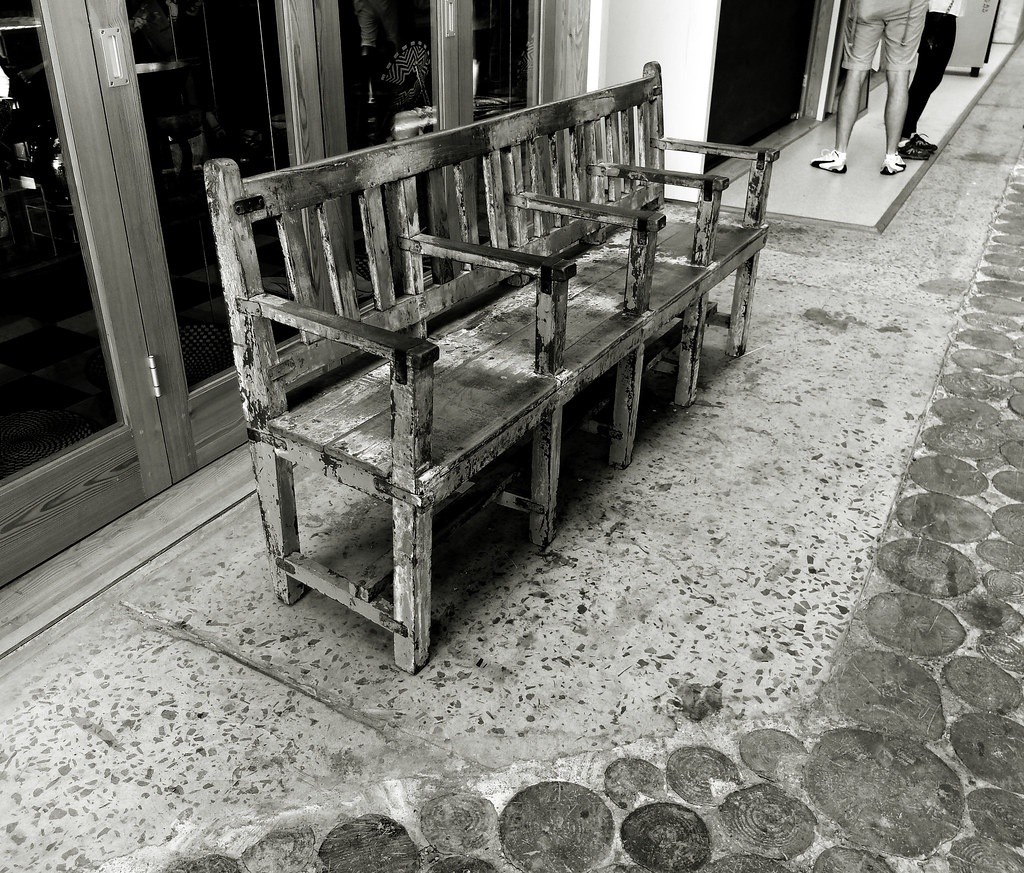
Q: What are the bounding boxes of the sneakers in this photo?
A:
[897,142,930,160]
[908,134,938,153]
[879,151,907,175]
[810,149,847,173]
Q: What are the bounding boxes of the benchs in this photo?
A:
[207,60,780,671]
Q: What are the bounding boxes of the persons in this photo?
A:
[895,0,968,160]
[810,0,927,175]
